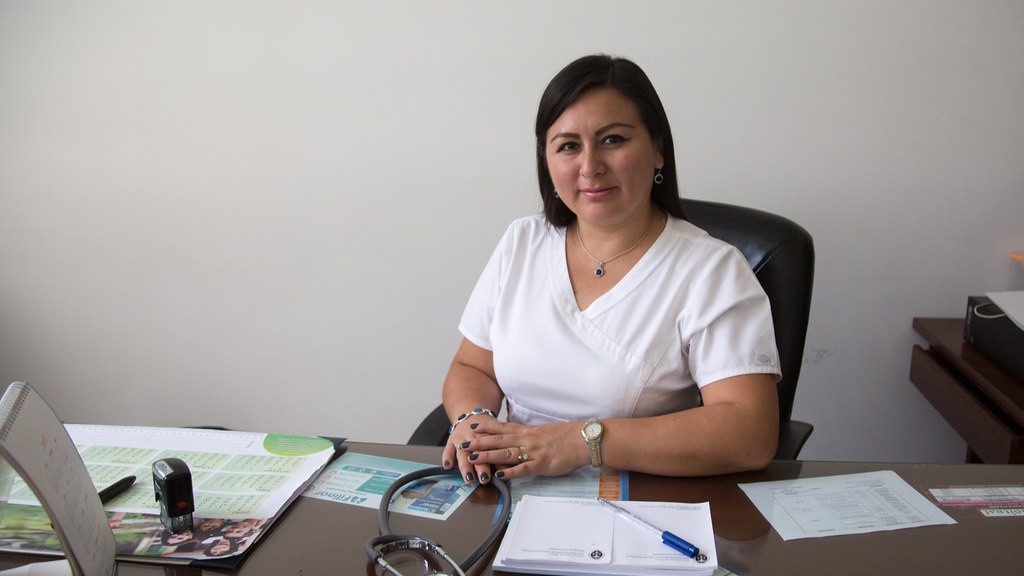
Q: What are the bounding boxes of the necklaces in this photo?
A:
[576,215,657,278]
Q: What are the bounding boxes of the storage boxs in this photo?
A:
[964,296,1024,384]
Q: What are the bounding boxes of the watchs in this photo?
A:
[580,417,603,468]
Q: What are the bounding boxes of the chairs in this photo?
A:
[402,196,816,469]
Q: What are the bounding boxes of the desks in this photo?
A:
[1,420,1024,576]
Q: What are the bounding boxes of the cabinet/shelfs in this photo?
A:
[908,314,1024,463]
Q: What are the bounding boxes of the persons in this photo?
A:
[442,56,783,484]
[160,517,268,557]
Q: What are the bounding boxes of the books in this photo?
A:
[492,492,719,575]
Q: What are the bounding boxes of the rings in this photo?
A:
[448,407,497,434]
[519,446,529,463]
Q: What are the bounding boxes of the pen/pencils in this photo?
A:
[98,475,137,504]
[594,495,699,558]
